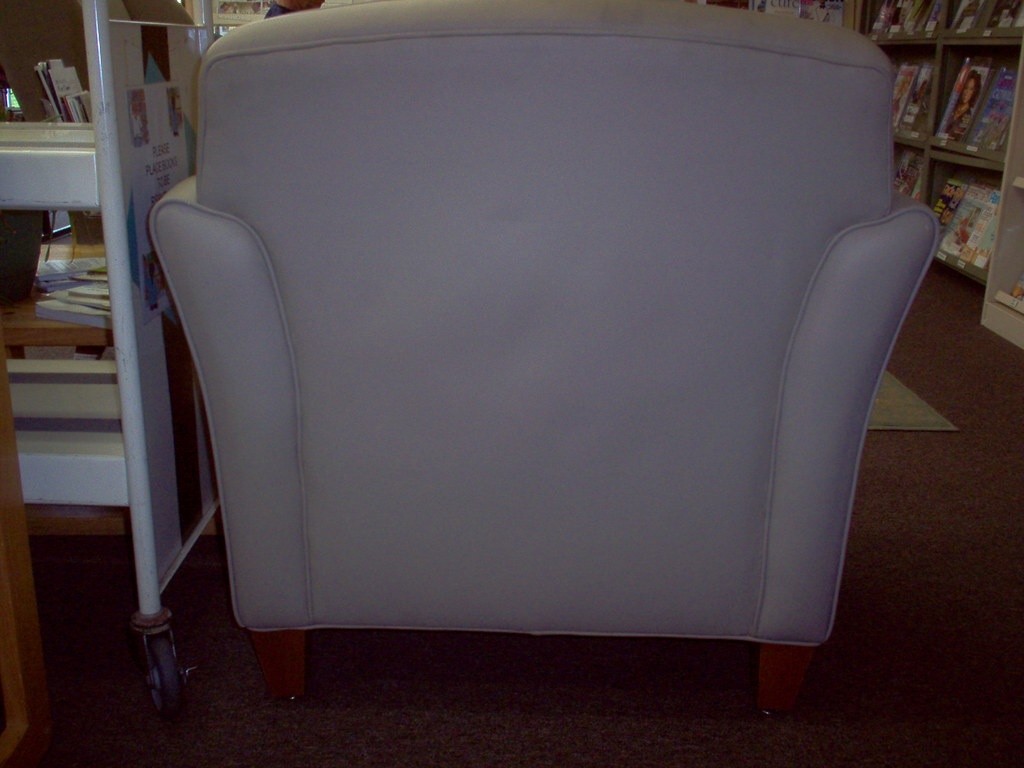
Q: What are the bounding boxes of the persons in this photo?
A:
[264,0,325,20]
[944,68,983,143]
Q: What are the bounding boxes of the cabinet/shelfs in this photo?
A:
[1,0,212,768]
[844,1,1024,353]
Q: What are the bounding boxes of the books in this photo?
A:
[924,176,1002,270]
[949,0,1024,31]
[869,0,943,34]
[32,256,113,331]
[935,52,1019,153]
[893,148,926,202]
[748,0,844,29]
[34,57,92,123]
[889,57,934,134]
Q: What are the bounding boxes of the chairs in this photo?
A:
[147,0,939,717]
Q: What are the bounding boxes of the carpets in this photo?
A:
[866,370,959,431]
